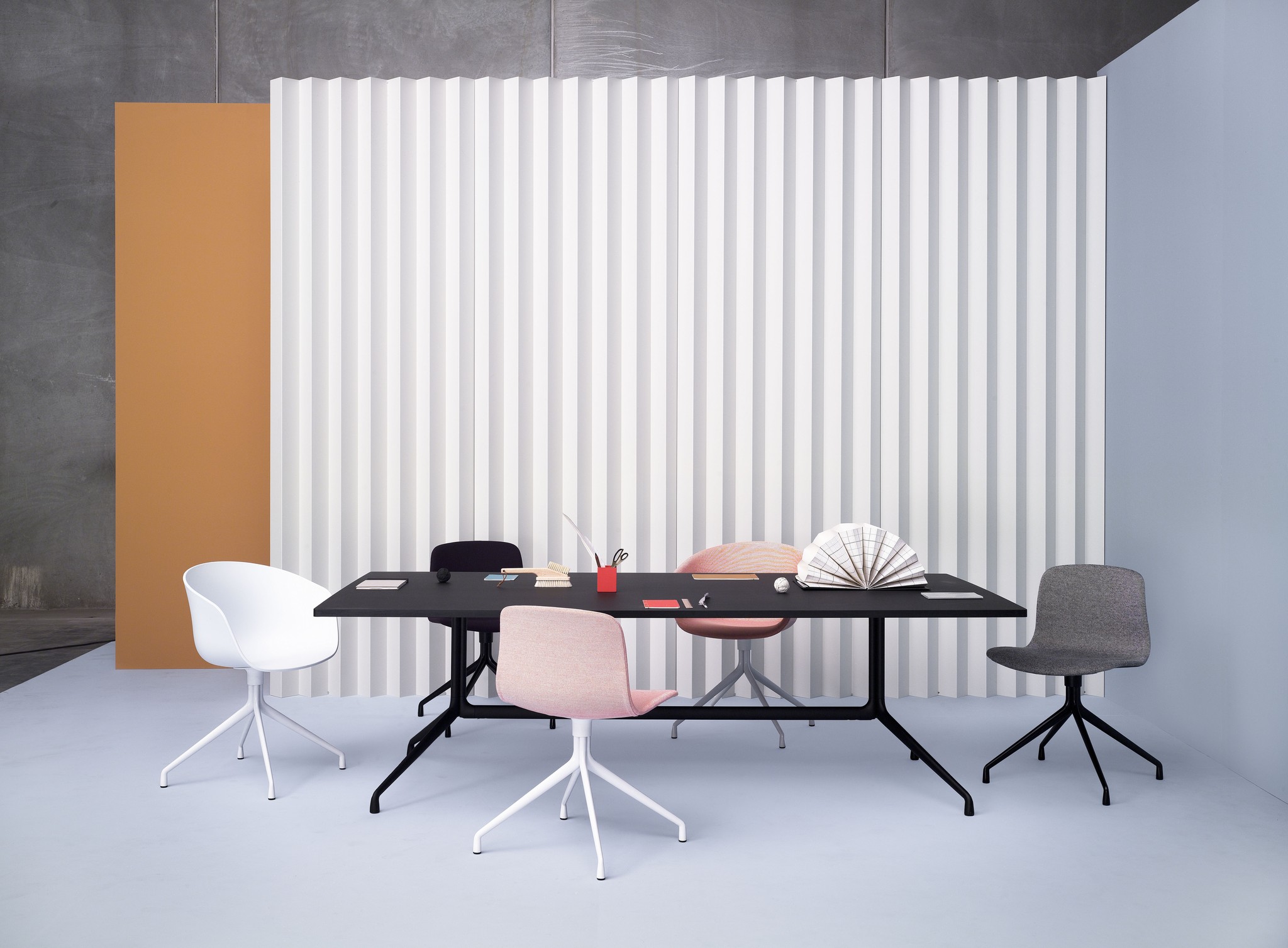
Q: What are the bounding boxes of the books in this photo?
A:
[484,573,519,581]
[795,524,930,590]
[921,592,983,599]
[642,599,680,609]
[356,578,409,590]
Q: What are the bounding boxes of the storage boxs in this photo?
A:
[597,565,617,592]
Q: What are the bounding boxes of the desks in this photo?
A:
[313,571,1028,818]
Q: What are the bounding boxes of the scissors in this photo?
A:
[611,549,629,568]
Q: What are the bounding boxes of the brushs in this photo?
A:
[501,561,572,587]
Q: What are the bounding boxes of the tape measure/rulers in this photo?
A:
[681,599,693,608]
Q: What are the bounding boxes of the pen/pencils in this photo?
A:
[563,513,606,568]
[595,553,601,568]
[698,592,711,608]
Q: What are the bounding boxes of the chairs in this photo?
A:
[671,541,814,750]
[470,603,688,880]
[411,540,556,740]
[982,565,1163,809]
[160,560,346,800]
